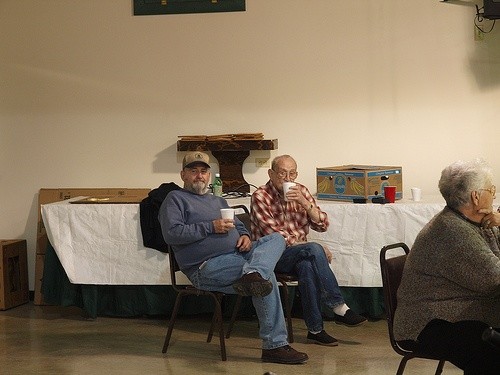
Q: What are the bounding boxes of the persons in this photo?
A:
[392,160,500,375]
[250,154,369,347]
[158,150,309,364]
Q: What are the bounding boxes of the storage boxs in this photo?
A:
[316,165,403,202]
[0,239,31,311]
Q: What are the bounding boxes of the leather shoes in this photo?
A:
[233,270,273,298]
[261,345,309,364]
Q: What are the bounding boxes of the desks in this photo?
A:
[41,196,444,322]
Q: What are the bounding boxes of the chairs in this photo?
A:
[380,243,446,375]
[158,205,299,361]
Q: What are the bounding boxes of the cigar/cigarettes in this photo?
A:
[332,256,336,259]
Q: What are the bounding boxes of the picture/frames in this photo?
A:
[133,0,246,16]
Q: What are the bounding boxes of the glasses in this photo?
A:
[479,184,497,196]
[272,168,298,180]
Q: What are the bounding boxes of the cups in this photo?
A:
[384,186,396,203]
[411,188,421,202]
[220,209,234,225]
[283,183,296,201]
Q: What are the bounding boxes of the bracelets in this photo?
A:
[306,204,313,212]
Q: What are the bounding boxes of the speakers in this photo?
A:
[483,0,500,20]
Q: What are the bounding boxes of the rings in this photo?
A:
[487,220,490,224]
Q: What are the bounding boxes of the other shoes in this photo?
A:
[333,307,369,327]
[306,330,339,346]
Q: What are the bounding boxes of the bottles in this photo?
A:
[213,174,222,197]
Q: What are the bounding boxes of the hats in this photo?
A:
[182,151,211,169]
